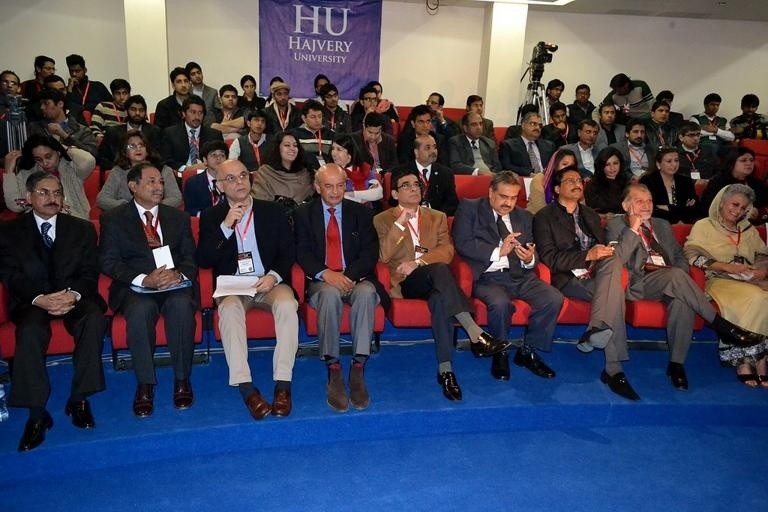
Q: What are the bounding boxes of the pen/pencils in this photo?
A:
[65,287,71,293]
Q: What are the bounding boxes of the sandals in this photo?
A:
[736,372,758,388]
[755,375,768,389]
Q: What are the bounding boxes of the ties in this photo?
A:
[423,169,428,180]
[189,130,199,164]
[640,223,670,265]
[212,180,219,198]
[327,207,343,271]
[40,223,54,248]
[471,140,477,149]
[658,129,665,145]
[497,214,524,282]
[528,142,541,174]
[144,212,161,248]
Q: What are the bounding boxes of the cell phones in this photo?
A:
[607,241,617,247]
[522,242,534,248]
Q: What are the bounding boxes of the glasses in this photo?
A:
[582,130,598,135]
[560,178,584,186]
[30,189,64,196]
[737,158,755,162]
[397,182,423,189]
[683,133,701,137]
[324,93,338,99]
[219,173,250,184]
[0,81,17,85]
[362,97,377,102]
[527,122,543,128]
[426,100,439,105]
[127,143,145,148]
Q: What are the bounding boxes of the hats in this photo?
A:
[271,82,290,93]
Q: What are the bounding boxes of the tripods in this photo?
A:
[517,65,549,126]
[5,108,27,152]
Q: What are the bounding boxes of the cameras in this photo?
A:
[529,42,558,64]
[5,94,30,107]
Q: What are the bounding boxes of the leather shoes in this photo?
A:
[576,327,614,353]
[471,333,511,358]
[601,369,640,401]
[721,325,765,345]
[437,370,462,401]
[273,387,292,417]
[18,413,53,452]
[491,352,510,380]
[245,388,272,420]
[350,366,369,410]
[66,398,93,428]
[174,382,193,409]
[133,391,154,417]
[513,348,555,378]
[327,368,349,413]
[667,365,688,392]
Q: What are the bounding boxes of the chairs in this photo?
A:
[536,262,629,325]
[83,171,101,219]
[374,259,473,328]
[382,173,395,211]
[83,110,91,127]
[290,260,390,337]
[752,225,768,246]
[454,175,492,202]
[516,175,532,210]
[492,127,508,146]
[198,266,306,341]
[294,102,306,110]
[622,266,706,329]
[98,217,213,352]
[104,171,111,181]
[395,106,414,131]
[1,208,14,220]
[226,139,233,149]
[694,179,709,199]
[182,165,206,194]
[149,112,155,125]
[441,108,468,123]
[448,217,551,326]
[0,284,75,359]
[740,137,768,180]
[671,224,720,315]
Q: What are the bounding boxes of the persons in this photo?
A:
[252,133,315,208]
[98,163,199,416]
[0,171,106,450]
[690,93,735,149]
[319,85,349,136]
[397,105,451,165]
[315,137,383,211]
[154,68,196,130]
[3,135,96,221]
[607,122,658,180]
[566,85,597,123]
[537,79,565,124]
[656,91,684,122]
[351,88,393,135]
[583,148,633,215]
[197,159,299,420]
[390,137,458,216]
[27,90,96,156]
[500,113,558,177]
[527,150,578,215]
[458,96,497,146]
[606,185,762,390]
[668,123,719,187]
[64,55,113,113]
[448,114,503,175]
[505,104,542,139]
[590,104,627,152]
[228,110,280,170]
[90,79,135,144]
[373,172,510,401]
[598,73,657,121]
[703,150,768,225]
[452,170,563,381]
[97,133,182,213]
[403,93,457,137]
[0,70,37,153]
[236,76,266,116]
[29,76,88,128]
[161,95,225,177]
[546,103,580,144]
[202,85,251,134]
[684,184,768,388]
[349,114,394,172]
[638,148,700,225]
[352,81,399,124]
[186,141,230,216]
[312,75,348,112]
[99,96,155,169]
[261,82,302,132]
[18,56,57,100]
[640,102,679,154]
[293,99,336,164]
[184,62,224,113]
[730,94,768,141]
[533,166,640,400]
[557,120,606,185]
[265,77,296,109]
[293,164,388,413]
